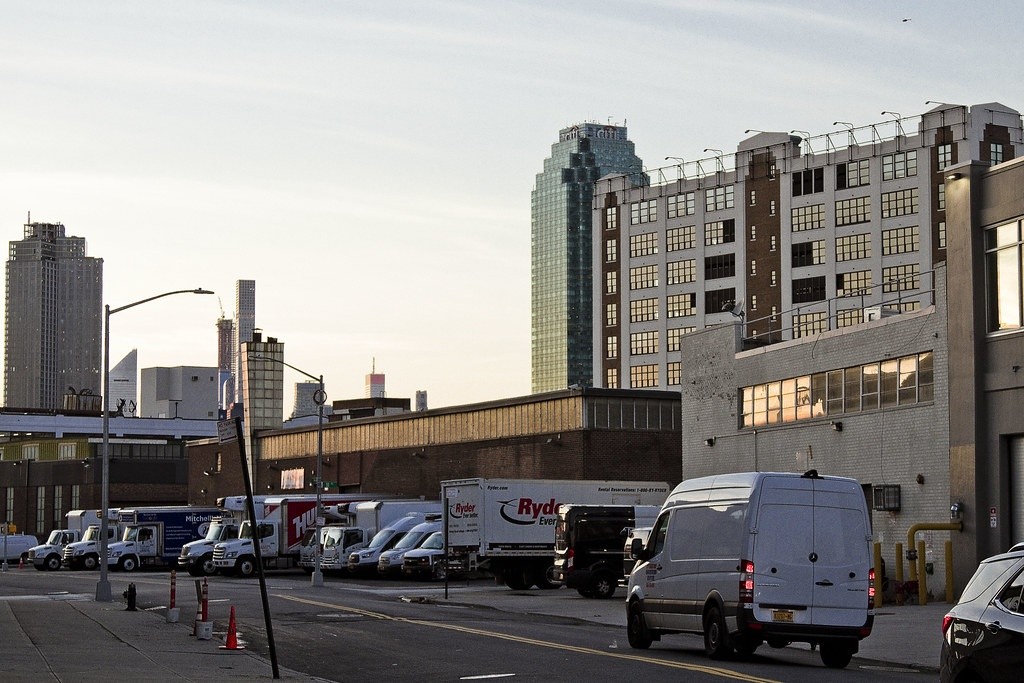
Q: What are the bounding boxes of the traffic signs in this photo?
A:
[217,419,239,445]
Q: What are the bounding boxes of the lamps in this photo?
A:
[829,421,843,432]
[203,470,214,478]
[944,171,962,182]
[703,436,716,447]
[411,451,422,460]
[81,459,89,465]
[13,461,21,467]
[546,438,559,447]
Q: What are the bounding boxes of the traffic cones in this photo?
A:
[218,605,246,649]
[188,599,203,636]
[18,557,25,570]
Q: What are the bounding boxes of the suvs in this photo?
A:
[941,540,1024,683]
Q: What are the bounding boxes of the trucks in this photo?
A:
[439,477,672,589]
[210,493,389,579]
[99,506,227,571]
[61,504,191,571]
[0,532,39,561]
[320,500,442,570]
[296,502,363,573]
[26,508,102,571]
[178,494,341,577]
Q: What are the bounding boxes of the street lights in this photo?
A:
[248,354,327,587]
[93,288,214,603]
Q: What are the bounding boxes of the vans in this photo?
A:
[551,501,664,599]
[347,512,430,574]
[625,467,875,670]
[401,529,467,582]
[377,512,442,571]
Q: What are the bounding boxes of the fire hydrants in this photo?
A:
[122,581,138,611]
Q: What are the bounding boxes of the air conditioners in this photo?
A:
[872,483,901,512]
